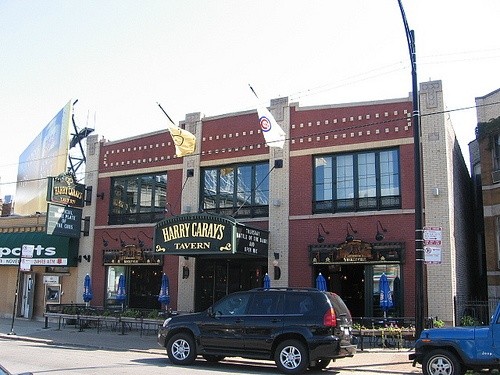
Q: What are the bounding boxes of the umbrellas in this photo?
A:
[379,272,393,320]
[83,274,92,308]
[158,273,170,312]
[263,273,270,290]
[116,273,127,311]
[316,272,327,291]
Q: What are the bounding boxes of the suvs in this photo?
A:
[158,288,357,374]
[407,299,500,375]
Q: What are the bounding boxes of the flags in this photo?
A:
[252,92,286,149]
[158,106,197,158]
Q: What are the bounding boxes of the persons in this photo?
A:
[47,288,53,296]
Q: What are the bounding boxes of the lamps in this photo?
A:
[346,222,357,243]
[102,231,118,248]
[137,231,153,248]
[375,220,388,241]
[317,223,330,243]
[119,231,136,248]
